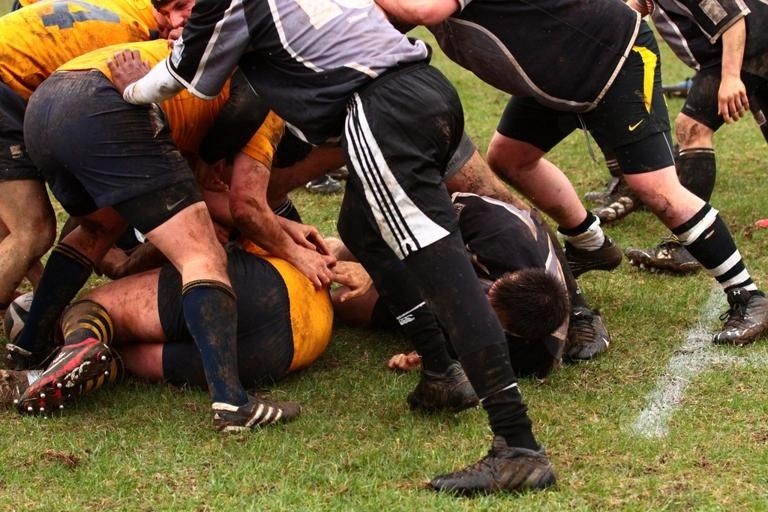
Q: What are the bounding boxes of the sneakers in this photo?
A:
[627,237,703,275]
[563,308,610,363]
[714,288,768,344]
[0,344,112,415]
[211,396,301,437]
[662,76,696,96]
[302,165,349,194]
[565,235,621,279]
[589,176,642,224]
[427,436,557,496]
[407,361,481,412]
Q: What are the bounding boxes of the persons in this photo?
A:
[582,171,639,224]
[622,0,767,278]
[101,0,564,498]
[0,0,611,419]
[374,0,767,349]
